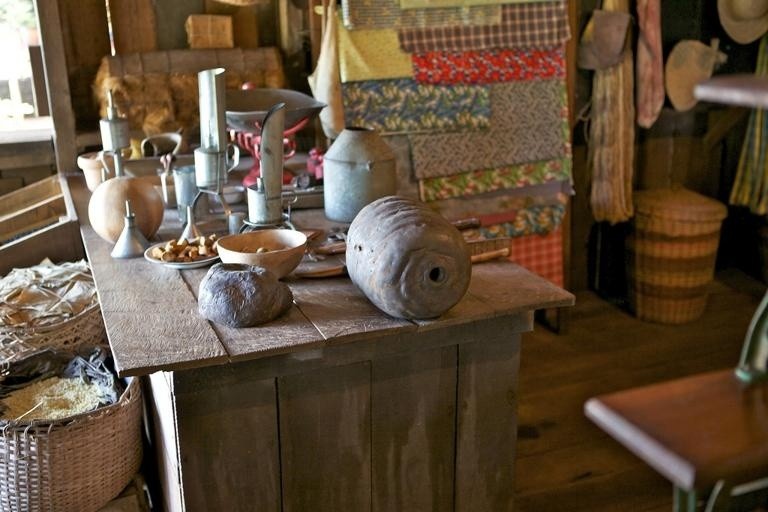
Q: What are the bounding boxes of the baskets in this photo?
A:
[0,344,146,512]
[626,184,729,326]
[1,258,109,379]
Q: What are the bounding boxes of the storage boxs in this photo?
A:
[0,172,86,279]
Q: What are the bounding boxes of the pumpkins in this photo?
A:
[88,176,164,245]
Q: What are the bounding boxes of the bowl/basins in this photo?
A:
[217,228,307,280]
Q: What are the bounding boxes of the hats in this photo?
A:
[717,0,768,45]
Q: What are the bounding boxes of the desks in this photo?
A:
[63,150,577,512]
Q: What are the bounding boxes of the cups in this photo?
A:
[247,184,298,224]
[77,152,115,193]
[194,143,239,187]
[173,167,196,221]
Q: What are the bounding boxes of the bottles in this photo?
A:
[98,106,130,151]
[323,126,396,223]
[110,214,151,259]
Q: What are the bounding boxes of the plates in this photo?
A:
[144,240,220,269]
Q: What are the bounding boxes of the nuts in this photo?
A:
[151,233,219,262]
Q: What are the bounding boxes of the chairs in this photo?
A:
[581,287,768,512]
